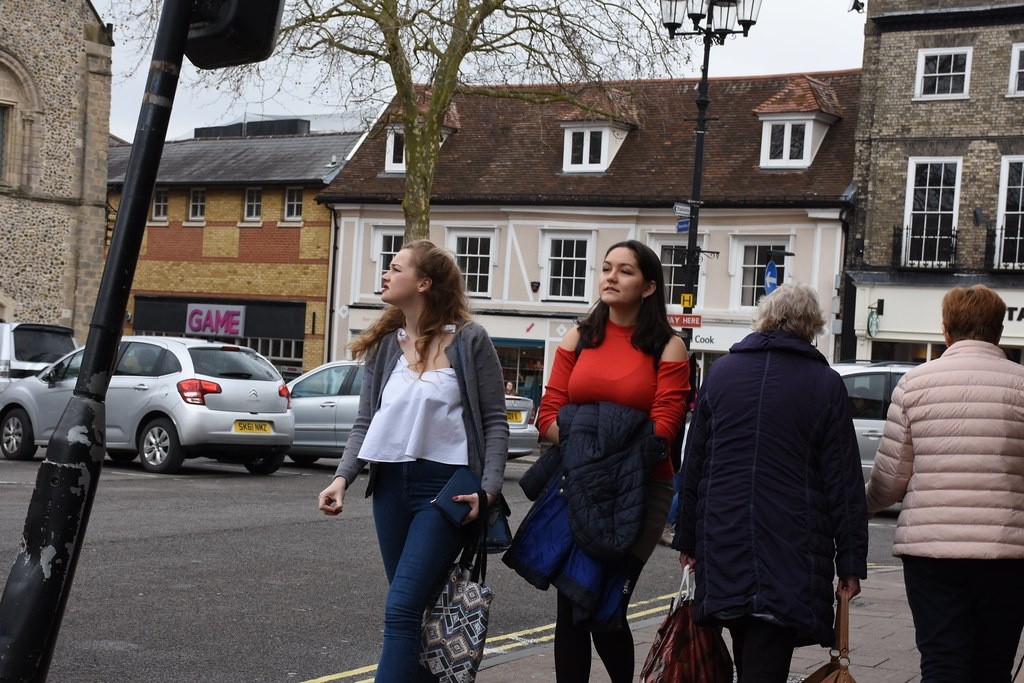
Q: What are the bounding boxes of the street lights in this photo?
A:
[660,0,760,471]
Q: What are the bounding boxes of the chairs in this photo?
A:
[139,349,157,374]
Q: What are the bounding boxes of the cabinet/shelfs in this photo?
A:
[502,355,544,382]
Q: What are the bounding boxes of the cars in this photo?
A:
[681,358,925,510]
[286,361,539,468]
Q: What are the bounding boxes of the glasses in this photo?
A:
[811,335,820,346]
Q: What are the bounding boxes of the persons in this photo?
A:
[319,241,510,683]
[534,240,690,683]
[505,382,516,396]
[671,285,867,683]
[865,286,1024,683]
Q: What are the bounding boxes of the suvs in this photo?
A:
[0,321,80,390]
[1,336,295,475]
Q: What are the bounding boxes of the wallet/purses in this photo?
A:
[431,469,480,523]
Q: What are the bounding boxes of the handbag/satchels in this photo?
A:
[640,567,733,683]
[801,589,856,683]
[416,489,495,682]
[468,494,514,555]
